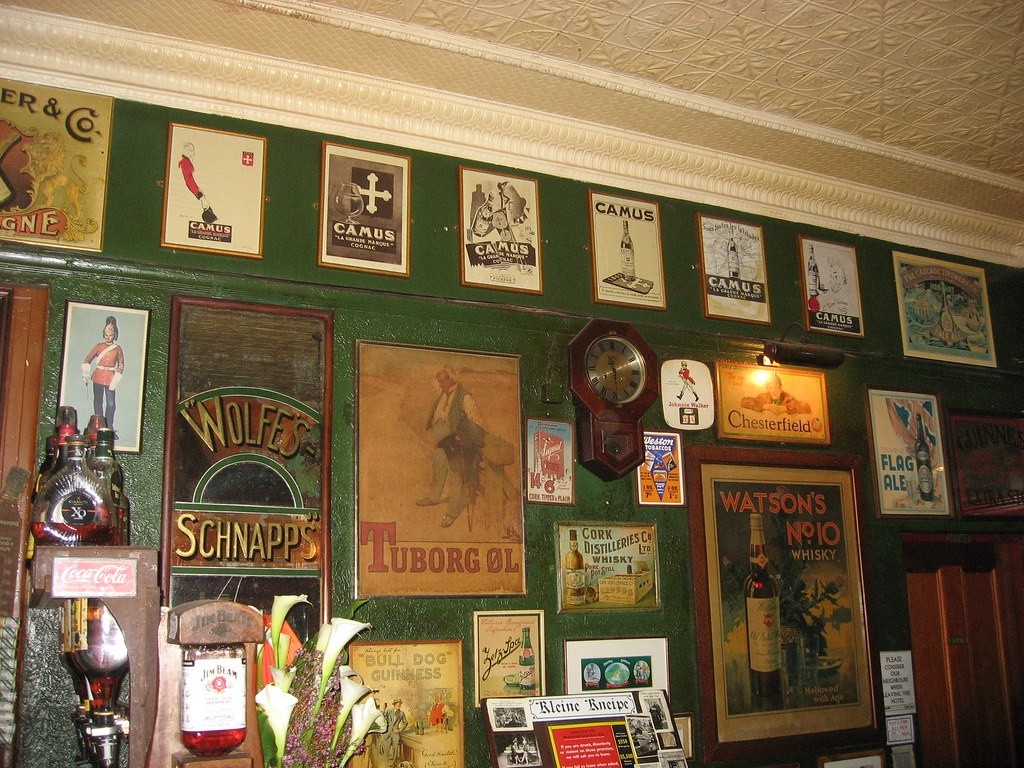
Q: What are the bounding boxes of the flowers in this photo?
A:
[246,594,388,768]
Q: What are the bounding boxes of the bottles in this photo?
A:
[31,434,119,547]
[111,470,130,545]
[26,435,56,570]
[89,428,114,495]
[38,406,77,492]
[59,598,129,768]
[179,645,246,757]
[82,415,107,460]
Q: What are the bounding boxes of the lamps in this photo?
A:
[763,322,845,369]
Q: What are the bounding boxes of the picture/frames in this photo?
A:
[472,609,546,708]
[673,712,696,762]
[350,340,529,600]
[685,446,887,767]
[892,250,997,368]
[798,233,865,339]
[817,749,886,768]
[317,140,411,279]
[562,635,671,704]
[552,520,662,614]
[56,299,152,455]
[347,637,465,767]
[861,384,956,520]
[696,211,772,326]
[587,188,667,312]
[715,360,831,445]
[458,165,544,296]
[160,121,268,259]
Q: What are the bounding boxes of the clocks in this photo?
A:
[568,318,661,482]
